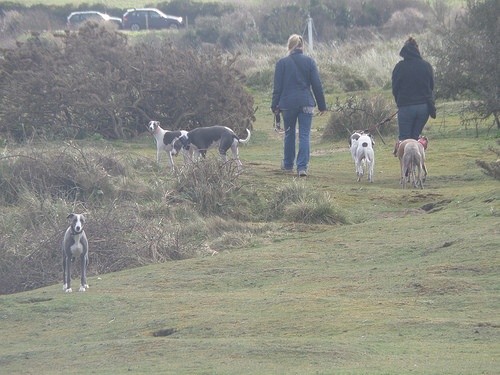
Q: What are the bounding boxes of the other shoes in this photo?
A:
[299,170,307,176]
[281,165,293,172]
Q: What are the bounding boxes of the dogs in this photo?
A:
[394,137,430,190]
[148,120,189,164]
[347,130,376,183]
[171,125,251,169]
[62,212,90,293]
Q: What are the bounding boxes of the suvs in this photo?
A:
[67,11,123,30]
[122,8,182,30]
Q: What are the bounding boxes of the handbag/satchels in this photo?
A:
[428,100,436,119]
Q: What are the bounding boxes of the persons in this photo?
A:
[392,37,435,178]
[272,33,326,175]
